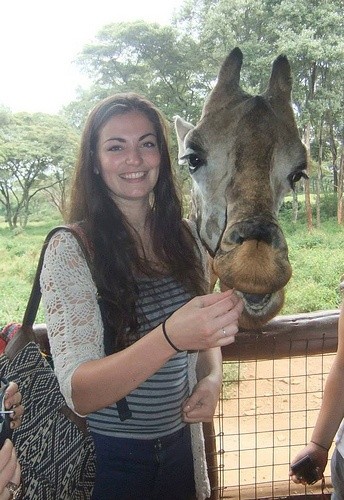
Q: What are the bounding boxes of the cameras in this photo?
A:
[290,454,323,485]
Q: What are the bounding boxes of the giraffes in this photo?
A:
[171,47,312,499]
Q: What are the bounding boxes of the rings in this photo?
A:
[223,328,227,338]
[310,441,330,452]
[6,482,23,500]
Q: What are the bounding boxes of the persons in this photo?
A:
[0,382,25,500]
[39,92,244,500]
[289,301,344,500]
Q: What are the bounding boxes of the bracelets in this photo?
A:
[162,320,185,353]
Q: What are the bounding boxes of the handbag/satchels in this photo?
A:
[0,226,101,500]
